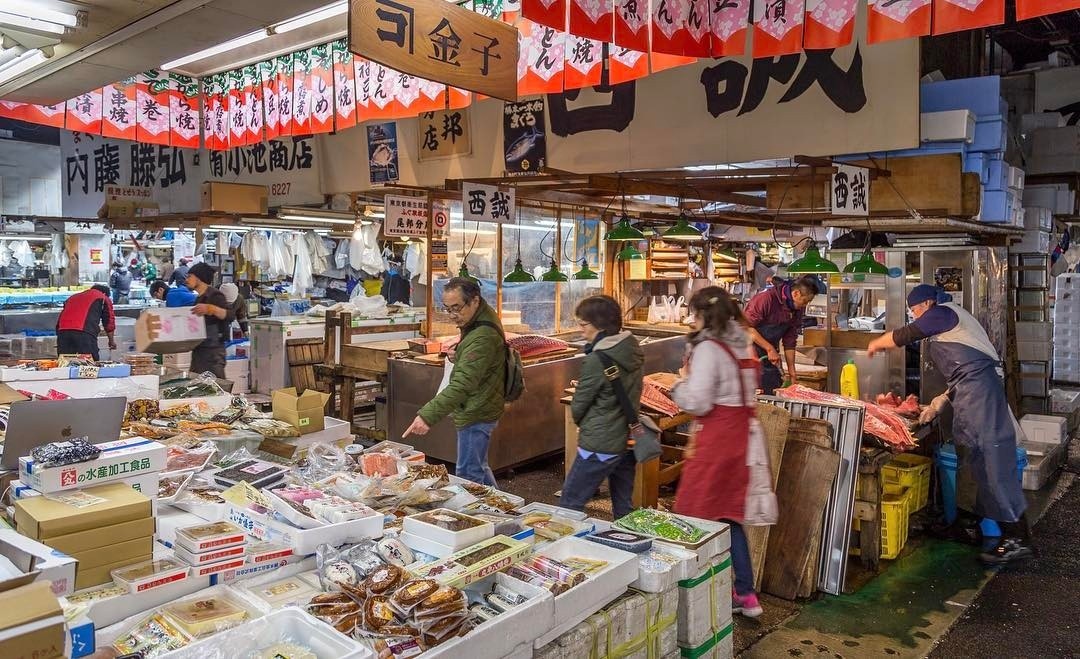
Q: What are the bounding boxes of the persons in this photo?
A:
[215,281,249,337]
[161,257,175,281]
[129,259,142,280]
[53,283,118,362]
[149,281,199,307]
[863,283,1036,563]
[109,261,131,304]
[402,276,511,491]
[182,262,237,379]
[168,258,189,287]
[140,255,157,285]
[558,293,646,527]
[380,254,409,307]
[741,277,820,398]
[669,284,780,617]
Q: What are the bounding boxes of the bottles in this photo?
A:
[840,360,860,400]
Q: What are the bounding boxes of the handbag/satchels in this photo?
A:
[628,416,663,464]
[506,343,524,402]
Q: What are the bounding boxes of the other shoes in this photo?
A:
[936,524,983,541]
[979,537,1039,564]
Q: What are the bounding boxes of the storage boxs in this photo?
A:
[0,351,736,658]
[846,443,1027,568]
[136,306,207,356]
[1006,181,1078,490]
[829,74,1025,228]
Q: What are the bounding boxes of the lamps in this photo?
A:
[716,242,738,263]
[841,176,890,276]
[662,195,703,240]
[539,203,568,283]
[503,199,535,283]
[615,243,644,260]
[451,200,482,287]
[0,0,351,99]
[243,204,385,236]
[785,166,840,274]
[604,184,645,240]
[570,208,599,280]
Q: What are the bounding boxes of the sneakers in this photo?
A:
[732,587,763,617]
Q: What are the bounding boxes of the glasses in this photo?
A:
[442,296,473,312]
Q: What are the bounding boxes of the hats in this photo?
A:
[219,283,238,304]
[188,262,214,285]
[907,284,936,308]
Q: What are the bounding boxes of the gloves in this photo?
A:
[931,394,949,414]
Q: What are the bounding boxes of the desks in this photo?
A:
[853,413,944,574]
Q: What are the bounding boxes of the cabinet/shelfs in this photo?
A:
[623,238,712,281]
[707,251,741,285]
